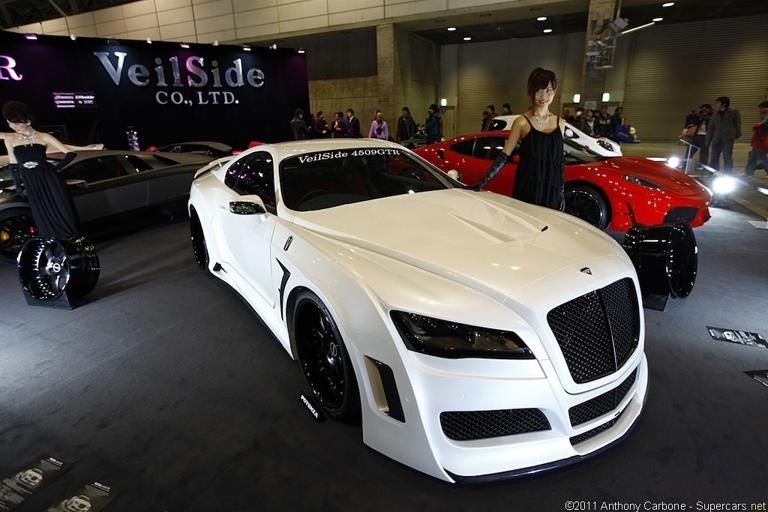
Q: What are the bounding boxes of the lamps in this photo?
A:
[25,32,305,54]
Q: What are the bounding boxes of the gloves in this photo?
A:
[466,152,508,191]
[559,177,567,212]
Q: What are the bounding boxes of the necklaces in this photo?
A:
[17,126,37,147]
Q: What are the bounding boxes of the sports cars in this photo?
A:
[188,134,653,489]
[387,129,712,230]
[0,141,233,263]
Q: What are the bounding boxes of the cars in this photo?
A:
[483,114,623,163]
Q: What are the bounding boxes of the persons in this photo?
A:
[4,102,77,242]
[683,97,768,176]
[291,103,641,144]
[460,68,565,212]
